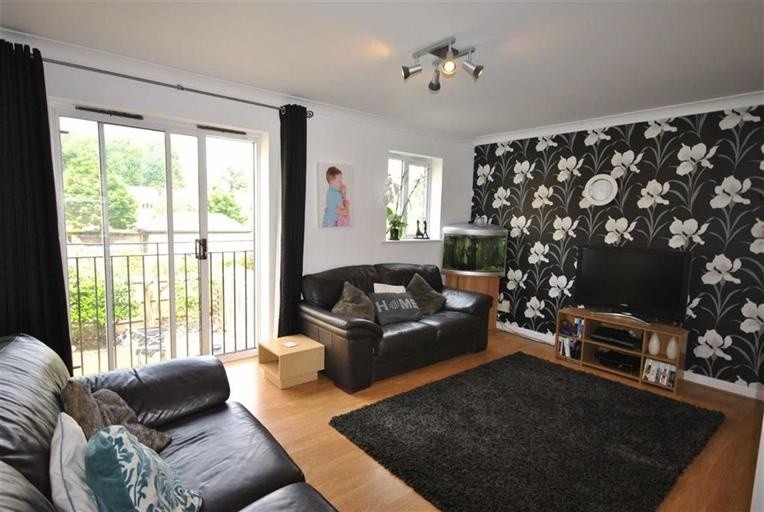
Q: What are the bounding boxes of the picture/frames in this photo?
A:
[316,162,354,230]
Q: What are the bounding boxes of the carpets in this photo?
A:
[330,350,726,512]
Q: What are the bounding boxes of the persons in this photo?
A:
[325,184,350,226]
[322,167,343,227]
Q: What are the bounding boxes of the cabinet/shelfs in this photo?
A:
[446,273,499,330]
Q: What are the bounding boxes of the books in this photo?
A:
[557,335,576,359]
[642,359,677,388]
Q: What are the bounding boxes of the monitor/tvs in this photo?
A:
[574,245,691,325]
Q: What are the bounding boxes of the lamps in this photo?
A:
[463,60,486,82]
[401,65,422,83]
[441,59,458,80]
[428,69,441,96]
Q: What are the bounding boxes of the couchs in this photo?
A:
[0,334,339,512]
[297,263,494,395]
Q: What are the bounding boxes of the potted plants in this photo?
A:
[386,208,408,239]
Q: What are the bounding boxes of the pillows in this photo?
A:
[84,425,203,512]
[368,291,423,326]
[406,273,446,315]
[61,377,172,453]
[331,281,376,323]
[49,412,98,512]
